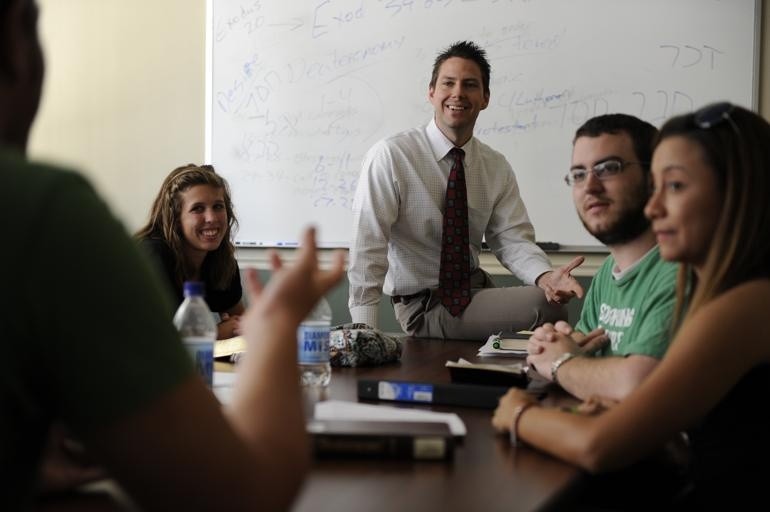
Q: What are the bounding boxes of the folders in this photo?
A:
[357,379,507,410]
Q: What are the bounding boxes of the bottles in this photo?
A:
[171,279,217,396]
[295,297,336,391]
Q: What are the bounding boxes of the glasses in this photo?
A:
[694,101,746,144]
[564,158,649,187]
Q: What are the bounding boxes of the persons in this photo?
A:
[2,0,346,512]
[525,112,697,413]
[344,39,585,340]
[491,101,770,512]
[129,162,247,340]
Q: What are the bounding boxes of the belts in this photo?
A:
[392,291,427,304]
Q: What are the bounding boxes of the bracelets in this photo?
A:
[550,352,579,388]
[508,400,538,450]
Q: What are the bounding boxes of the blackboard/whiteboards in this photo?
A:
[202,0,765,257]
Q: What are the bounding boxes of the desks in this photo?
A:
[294,334,583,512]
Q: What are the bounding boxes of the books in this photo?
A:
[295,416,457,468]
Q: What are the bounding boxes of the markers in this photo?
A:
[235,241,263,245]
[277,241,299,246]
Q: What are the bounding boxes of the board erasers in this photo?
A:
[535,241,561,250]
[480,242,489,249]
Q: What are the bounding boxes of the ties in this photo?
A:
[438,147,471,320]
